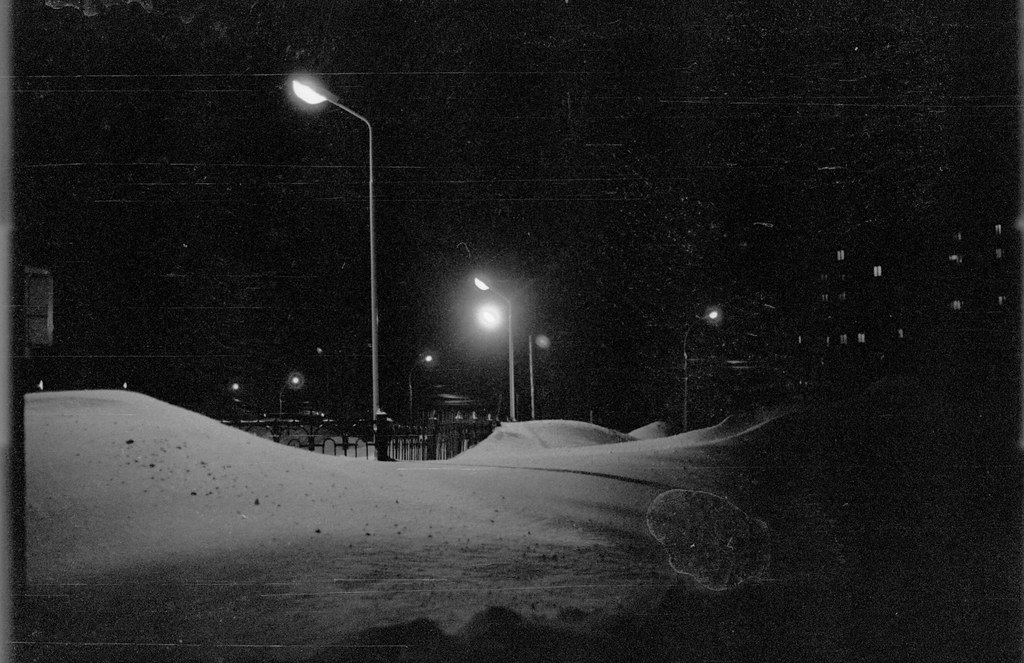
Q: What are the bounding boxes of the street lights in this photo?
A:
[682,309,718,432]
[475,277,514,422]
[289,77,380,461]
[409,354,433,425]
